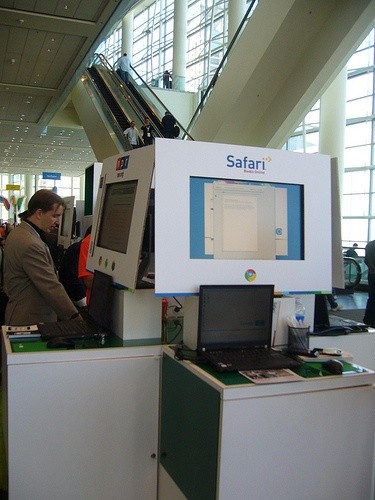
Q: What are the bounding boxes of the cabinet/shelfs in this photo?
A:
[0,324,375,500]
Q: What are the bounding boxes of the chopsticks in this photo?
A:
[286,311,309,328]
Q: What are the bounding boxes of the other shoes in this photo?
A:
[331,304,342,312]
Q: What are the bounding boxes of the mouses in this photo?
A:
[46,338,75,349]
[321,359,347,375]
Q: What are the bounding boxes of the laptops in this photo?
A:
[196,285,297,372]
[36,269,113,341]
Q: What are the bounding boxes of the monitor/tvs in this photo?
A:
[190,176,305,260]
[96,179,137,254]
[60,207,71,238]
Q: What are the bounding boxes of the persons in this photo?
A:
[168,126,181,138]
[328,294,343,311]
[140,117,155,146]
[58,224,94,305]
[161,111,175,134]
[162,70,172,88]
[115,53,132,84]
[123,121,140,149]
[2,189,82,326]
[0,222,19,247]
[365,240,375,329]
[346,243,358,256]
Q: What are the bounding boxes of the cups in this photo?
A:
[288,325,310,353]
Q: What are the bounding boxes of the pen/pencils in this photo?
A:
[286,318,308,351]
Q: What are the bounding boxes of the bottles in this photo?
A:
[293,297,305,325]
